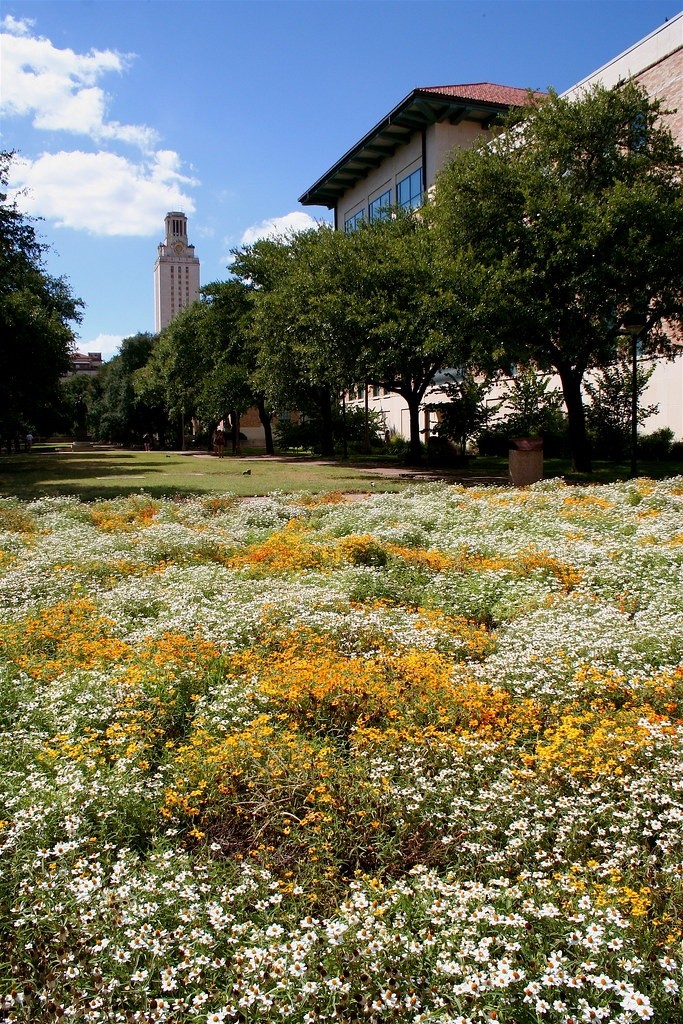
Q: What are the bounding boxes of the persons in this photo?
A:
[214,426,225,458]
[143,433,151,452]
[385,430,390,442]
[27,433,33,448]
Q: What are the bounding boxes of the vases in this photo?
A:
[510,438,544,451]
